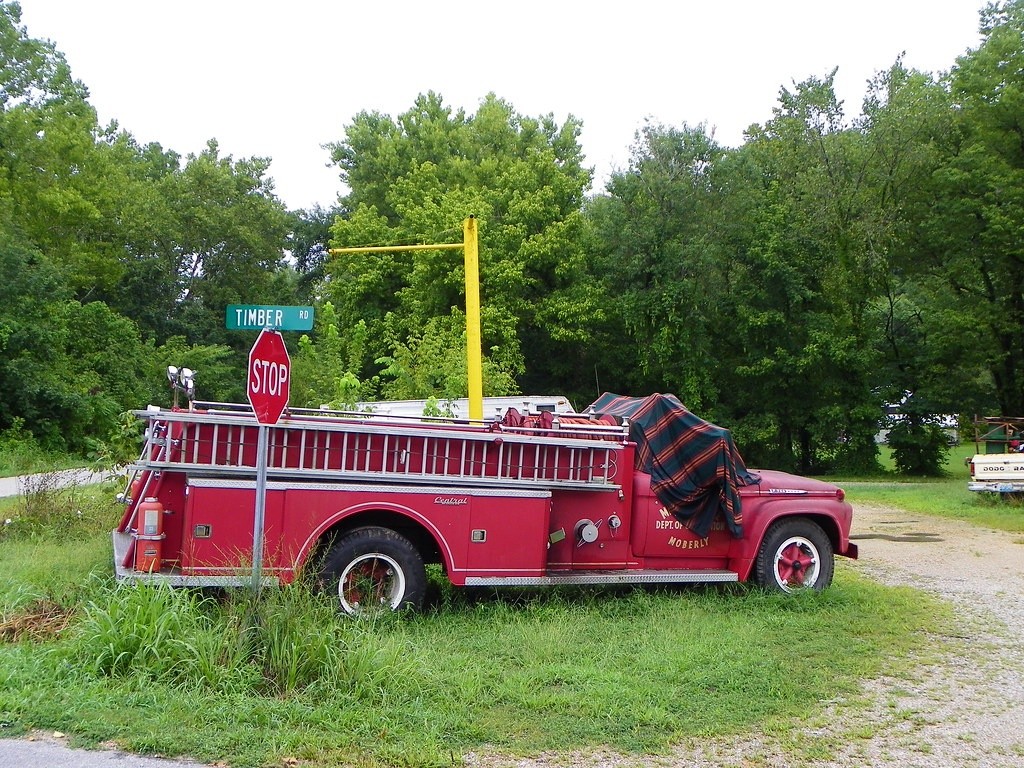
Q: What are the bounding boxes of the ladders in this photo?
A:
[125,407,625,493]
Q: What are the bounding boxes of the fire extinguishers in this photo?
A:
[136,497,164,573]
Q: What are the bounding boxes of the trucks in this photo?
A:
[965,452,1024,495]
[114,365,859,630]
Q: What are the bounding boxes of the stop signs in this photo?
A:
[246,328,291,428]
